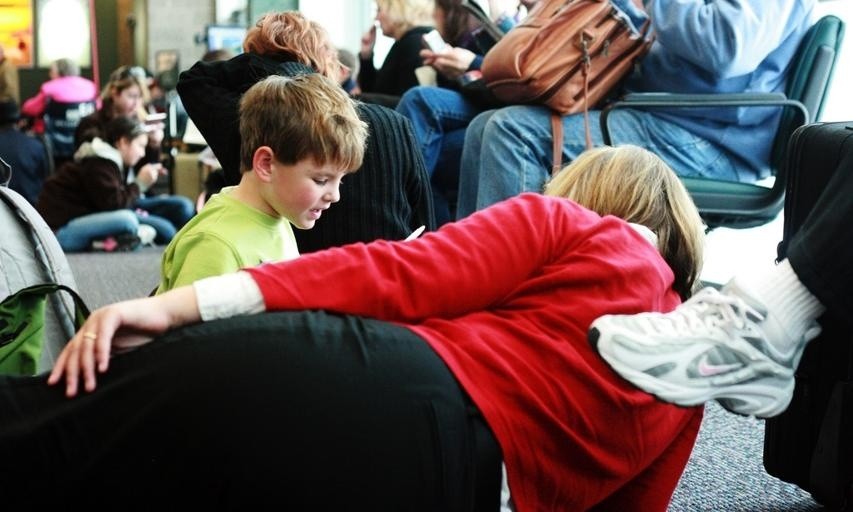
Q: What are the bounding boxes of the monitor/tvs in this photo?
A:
[205,24,247,58]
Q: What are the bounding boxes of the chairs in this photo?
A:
[138,100,180,196]
[43,110,77,176]
[599,12,845,238]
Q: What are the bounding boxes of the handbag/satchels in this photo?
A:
[480,0,657,115]
[0,281,94,377]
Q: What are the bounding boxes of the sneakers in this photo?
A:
[587,285,798,420]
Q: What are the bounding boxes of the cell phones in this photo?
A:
[402,225,426,242]
